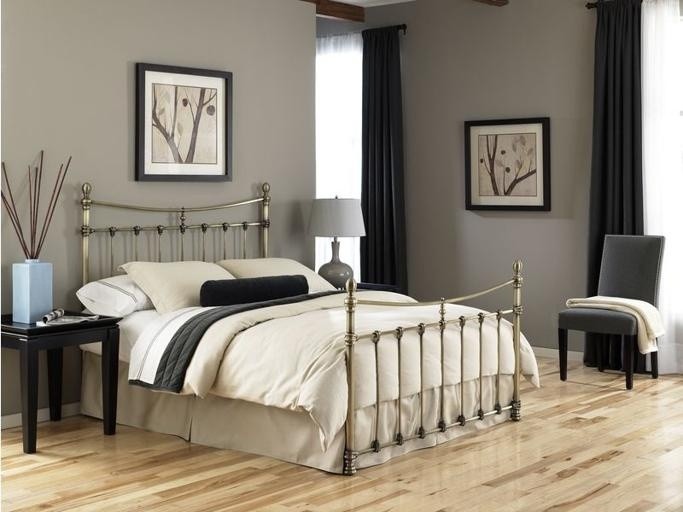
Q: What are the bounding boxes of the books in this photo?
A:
[36,308,100,325]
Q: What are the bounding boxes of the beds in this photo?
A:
[77,183,523,475]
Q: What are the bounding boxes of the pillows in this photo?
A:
[76,258,338,318]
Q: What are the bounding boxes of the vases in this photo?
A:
[11,259,53,325]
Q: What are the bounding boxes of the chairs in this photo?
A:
[558,234,666,390]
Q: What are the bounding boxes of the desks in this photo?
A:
[1,310,124,454]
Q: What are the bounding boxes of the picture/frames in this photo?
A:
[134,62,233,183]
[464,117,551,212]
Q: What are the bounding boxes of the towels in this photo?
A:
[565,296,666,355]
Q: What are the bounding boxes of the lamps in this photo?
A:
[307,196,367,290]
